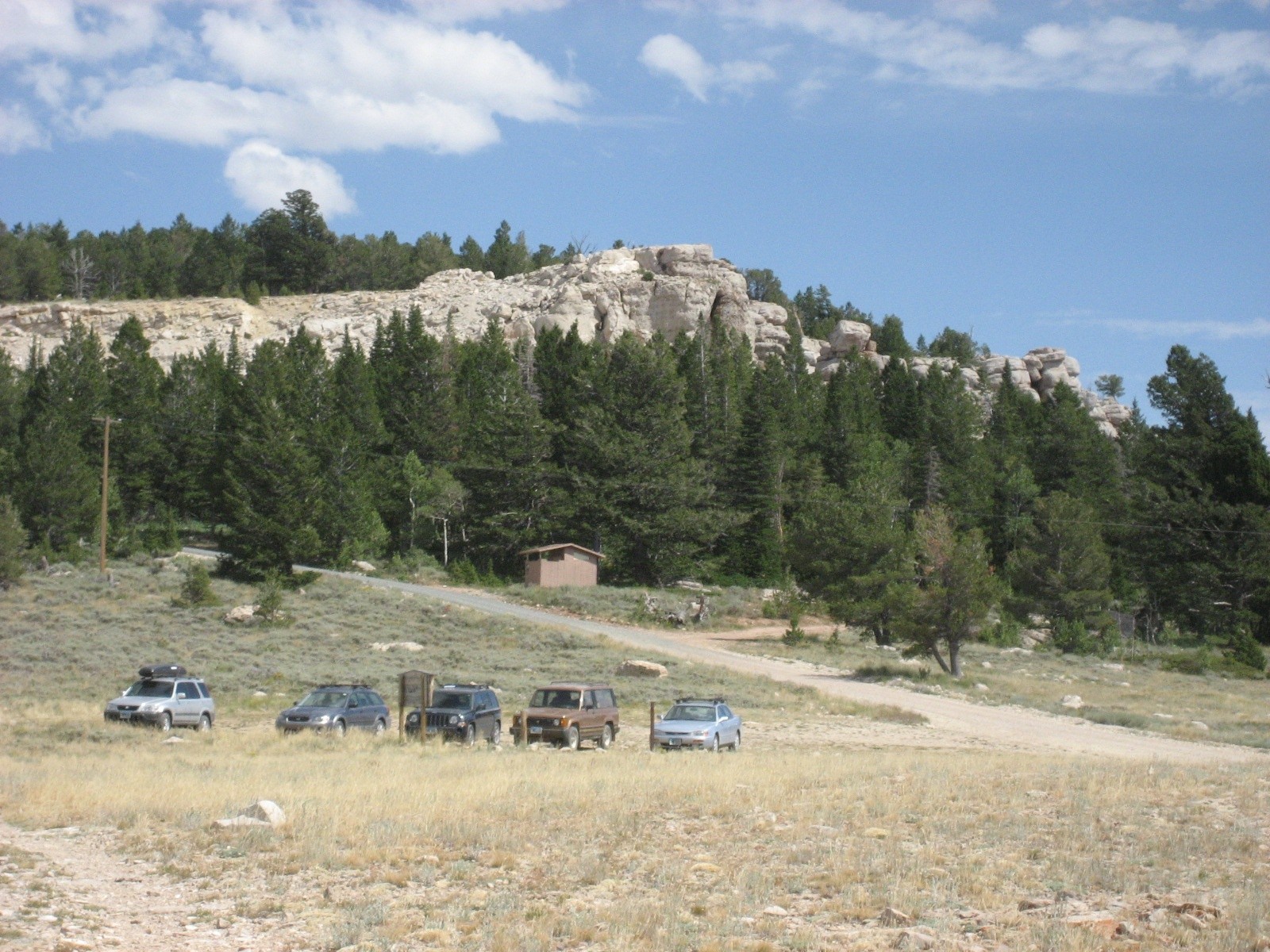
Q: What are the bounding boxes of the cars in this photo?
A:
[652,698,746,752]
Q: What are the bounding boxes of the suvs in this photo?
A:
[508,680,621,750]
[403,683,503,746]
[272,684,391,736]
[102,663,216,735]
